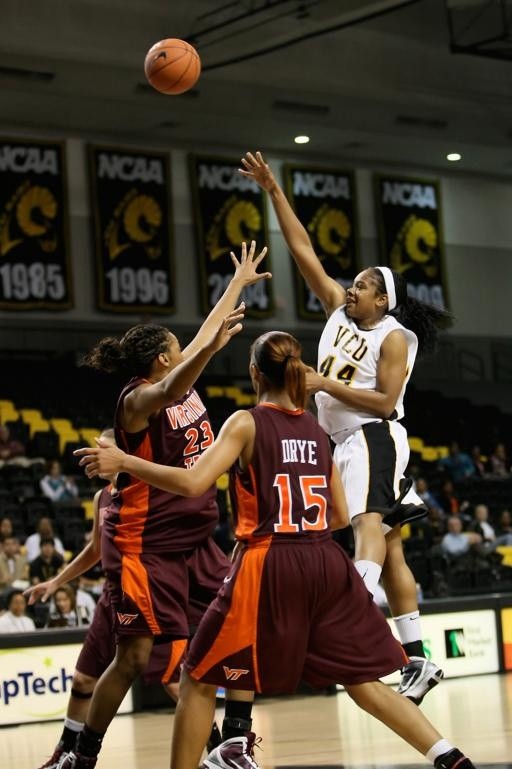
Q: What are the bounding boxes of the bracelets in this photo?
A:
[267,184,279,196]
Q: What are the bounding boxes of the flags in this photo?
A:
[0,133,77,315]
[280,161,366,323]
[187,151,277,319]
[369,168,454,330]
[82,140,182,316]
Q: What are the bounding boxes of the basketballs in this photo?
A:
[144,38,200,95]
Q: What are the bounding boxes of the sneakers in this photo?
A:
[398,656,443,707]
[202,731,262,769]
[36,741,98,769]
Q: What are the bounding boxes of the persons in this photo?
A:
[51,240,272,769]
[72,330,477,767]
[238,149,448,705]
[19,426,221,768]
[1,417,512,631]
